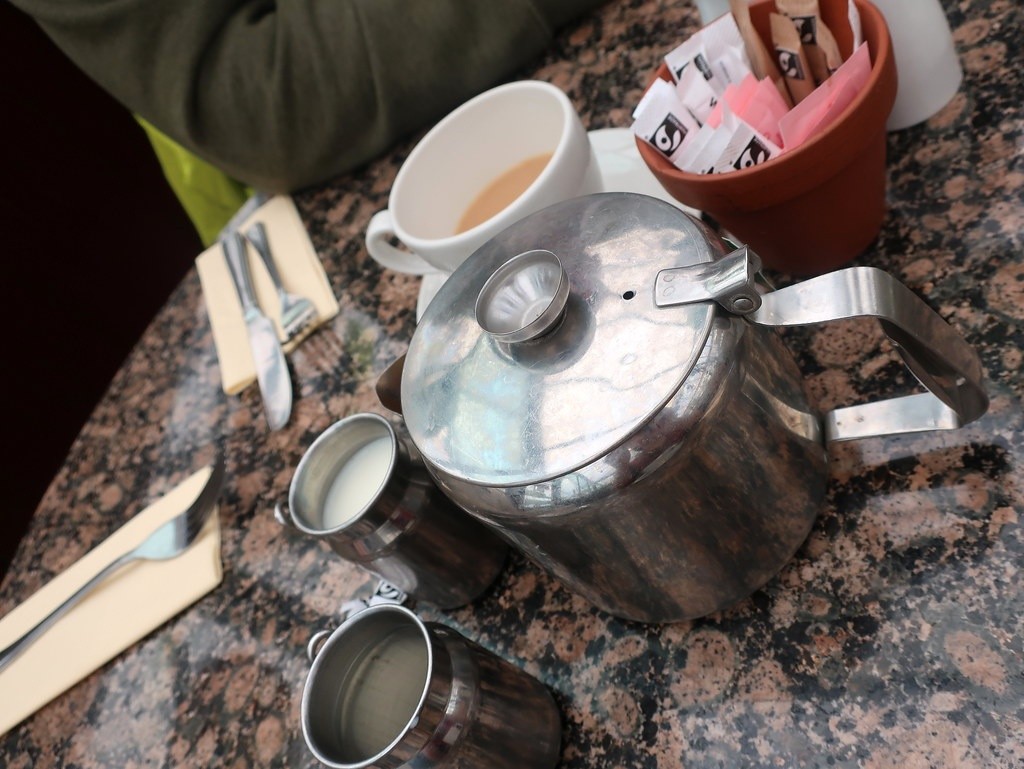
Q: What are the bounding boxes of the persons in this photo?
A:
[2,0,617,251]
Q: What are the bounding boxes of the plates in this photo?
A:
[417,125,703,335]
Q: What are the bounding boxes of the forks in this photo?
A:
[244,222,352,377]
[0,451,228,676]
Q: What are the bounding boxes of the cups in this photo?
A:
[299,604,563,769]
[275,414,507,609]
[365,83,609,273]
[636,1,899,279]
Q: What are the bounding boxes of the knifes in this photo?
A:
[223,231,293,432]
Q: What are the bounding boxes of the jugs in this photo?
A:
[373,190,992,626]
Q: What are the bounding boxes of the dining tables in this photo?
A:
[0,0,1022,769]
